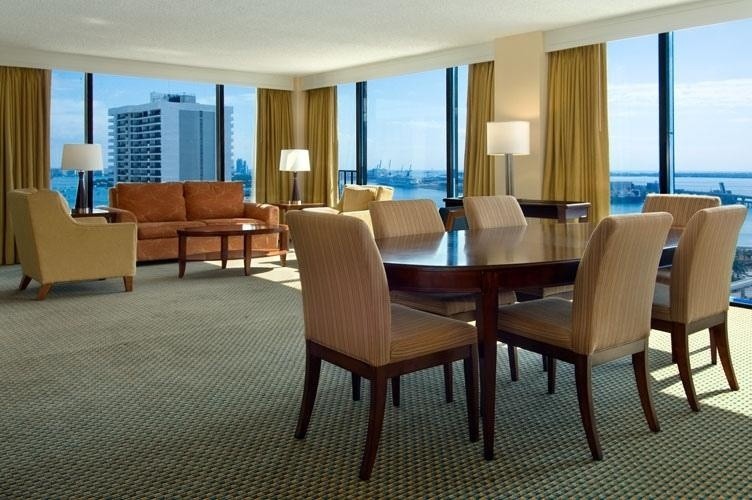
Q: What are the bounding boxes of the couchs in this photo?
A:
[9,188,138,299]
[105,182,280,262]
[303,183,394,242]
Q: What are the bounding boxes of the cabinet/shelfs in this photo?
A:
[443,197,592,222]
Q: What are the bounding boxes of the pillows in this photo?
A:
[340,186,376,214]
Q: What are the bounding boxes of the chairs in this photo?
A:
[640,194,720,230]
[463,195,575,394]
[368,199,518,403]
[493,212,671,461]
[284,211,480,482]
[651,204,748,413]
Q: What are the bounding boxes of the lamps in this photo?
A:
[486,121,530,195]
[61,143,103,215]
[279,149,312,206]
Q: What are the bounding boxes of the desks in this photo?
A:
[70,208,112,220]
[272,201,324,226]
[374,219,687,459]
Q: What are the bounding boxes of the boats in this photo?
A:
[48,166,115,211]
[366,160,469,199]
[608,175,751,217]
[230,158,257,203]
